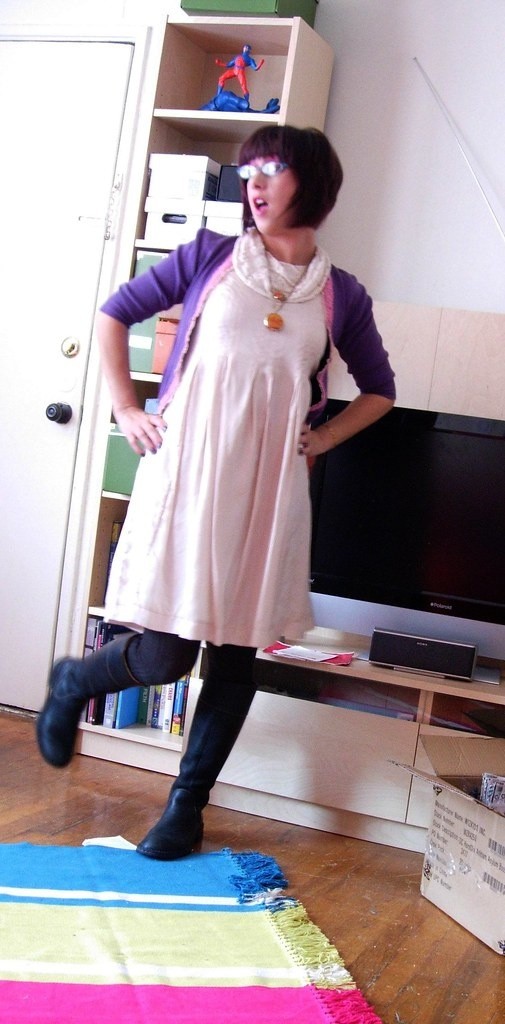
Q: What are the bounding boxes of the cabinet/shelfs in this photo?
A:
[61,15,335,777]
[405,663,505,853]
[196,638,426,853]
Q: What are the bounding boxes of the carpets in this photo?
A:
[0,843,380,1024]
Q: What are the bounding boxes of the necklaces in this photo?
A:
[263,244,318,330]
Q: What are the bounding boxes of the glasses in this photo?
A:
[236,161,293,182]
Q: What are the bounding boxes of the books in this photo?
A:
[103,520,122,612]
[80,613,186,741]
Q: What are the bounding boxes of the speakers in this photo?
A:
[369,627,478,680]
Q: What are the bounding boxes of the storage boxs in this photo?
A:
[157,302,182,322]
[215,164,241,202]
[102,423,140,496]
[201,201,244,237]
[133,250,168,280]
[152,321,177,375]
[141,198,206,251]
[180,0,317,29]
[389,733,505,956]
[147,153,220,202]
[127,314,155,373]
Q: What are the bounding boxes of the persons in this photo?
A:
[215,45,265,105]
[38,128,397,861]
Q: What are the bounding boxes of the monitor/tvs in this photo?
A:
[308,397,505,684]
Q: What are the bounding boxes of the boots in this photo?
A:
[34,633,144,767]
[136,693,248,860]
